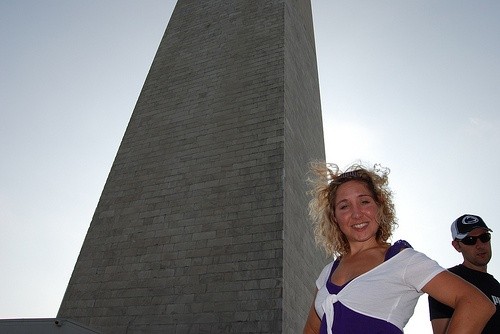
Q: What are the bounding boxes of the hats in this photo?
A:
[451,214,493,241]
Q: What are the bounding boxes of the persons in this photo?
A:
[301,161,496,334]
[428,214,500,334]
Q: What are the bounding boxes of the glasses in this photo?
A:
[456,232,491,245]
